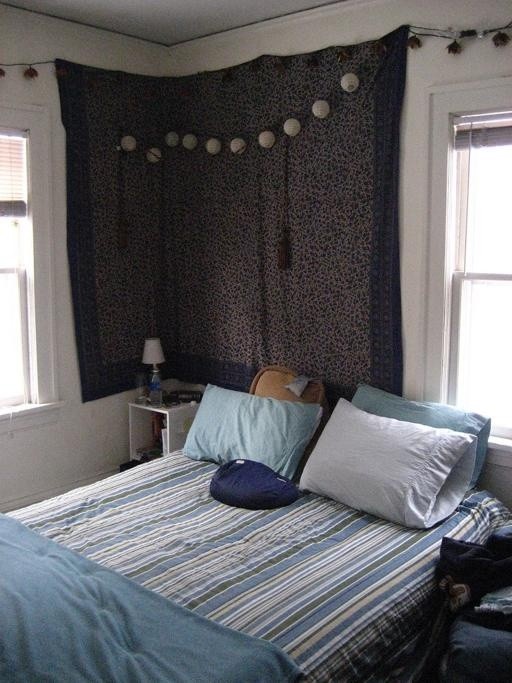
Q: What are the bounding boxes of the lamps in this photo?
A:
[141,337,166,390]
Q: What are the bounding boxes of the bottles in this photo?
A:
[150,369,162,407]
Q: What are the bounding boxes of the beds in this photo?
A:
[0,447,496,683]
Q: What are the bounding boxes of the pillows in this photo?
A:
[351,382,490,488]
[210,457,297,509]
[181,382,322,479]
[180,366,491,530]
[248,368,327,422]
[298,397,478,528]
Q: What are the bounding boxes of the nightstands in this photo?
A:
[128,400,202,462]
